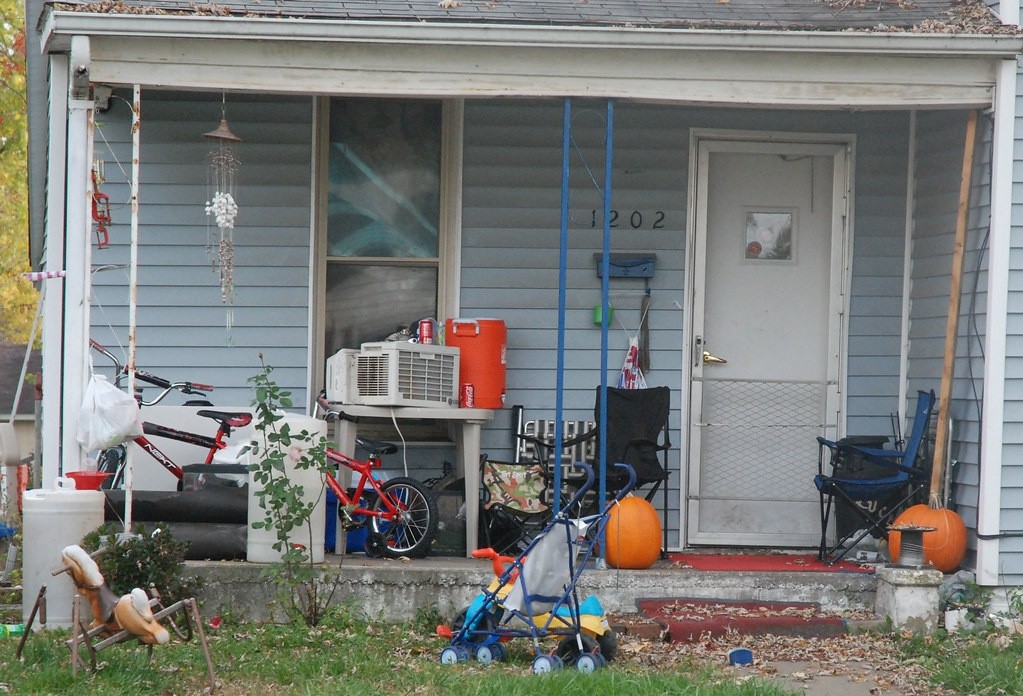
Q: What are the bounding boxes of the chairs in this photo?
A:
[474,454,599,557]
[811,388,937,567]
[511,383,672,560]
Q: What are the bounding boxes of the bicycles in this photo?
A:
[86,335,254,498]
[232,387,440,560]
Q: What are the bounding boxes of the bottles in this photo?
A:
[0,623,37,639]
[846,549,882,562]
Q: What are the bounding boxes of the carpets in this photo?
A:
[634,596,846,664]
[665,551,876,576]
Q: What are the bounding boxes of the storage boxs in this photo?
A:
[324,486,410,556]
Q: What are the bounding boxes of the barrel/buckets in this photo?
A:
[22,477,105,631]
[593,304,613,326]
[445,317,507,409]
[246,412,327,564]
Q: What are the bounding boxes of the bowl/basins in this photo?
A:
[65,471,108,490]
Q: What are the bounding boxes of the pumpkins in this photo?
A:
[888,494,968,574]
[597,491,663,568]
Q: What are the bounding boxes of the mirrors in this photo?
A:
[740,204,799,266]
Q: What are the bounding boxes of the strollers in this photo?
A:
[434,461,637,673]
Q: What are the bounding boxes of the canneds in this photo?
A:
[461,383,474,408]
[420,320,433,345]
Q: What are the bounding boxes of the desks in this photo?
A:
[327,403,495,558]
